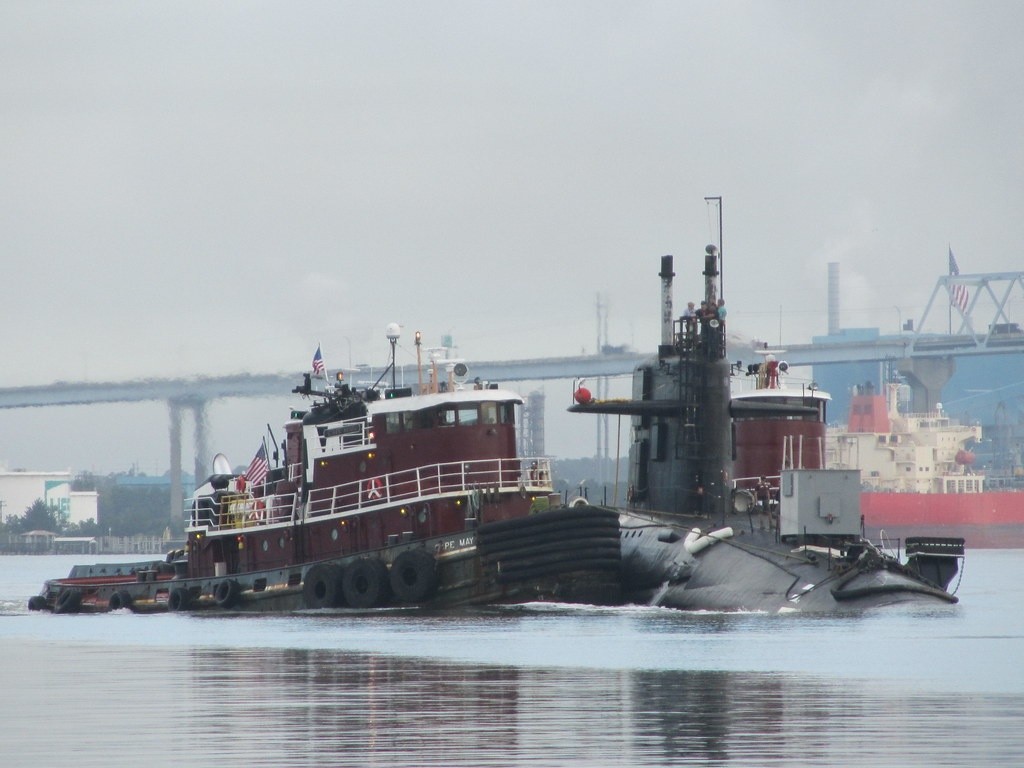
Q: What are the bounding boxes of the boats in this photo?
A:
[32,196,972,619]
[807,349,1023,548]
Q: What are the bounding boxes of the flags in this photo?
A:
[244,443,270,484]
[947,247,971,320]
[311,346,325,376]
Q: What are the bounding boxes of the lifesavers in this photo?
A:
[249,501,264,520]
[367,478,383,499]
